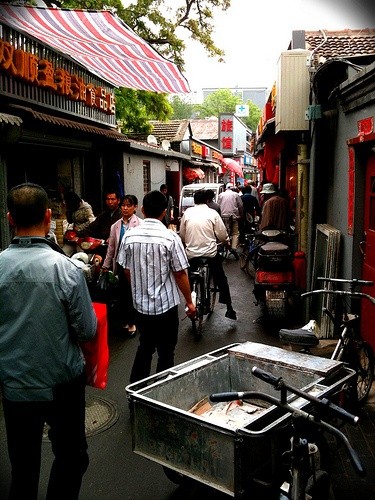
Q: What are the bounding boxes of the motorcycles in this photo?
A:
[253,231,295,328]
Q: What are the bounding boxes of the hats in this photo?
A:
[260,183,275,194]
[226,183,233,189]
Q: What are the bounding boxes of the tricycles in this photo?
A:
[124,275,375,500]
[209,364,362,500]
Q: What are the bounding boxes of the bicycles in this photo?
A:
[225,217,257,272]
[186,241,224,339]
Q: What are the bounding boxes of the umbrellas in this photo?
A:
[221,157,244,178]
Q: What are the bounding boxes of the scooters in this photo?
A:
[64,234,121,338]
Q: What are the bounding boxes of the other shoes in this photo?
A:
[225,311,236,321]
[120,326,137,338]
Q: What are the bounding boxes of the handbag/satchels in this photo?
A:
[85,302,109,389]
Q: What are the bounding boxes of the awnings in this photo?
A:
[183,167,205,179]
[0,3,192,94]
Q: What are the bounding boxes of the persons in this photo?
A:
[160,184,174,228]
[180,191,236,320]
[235,182,243,196]
[78,187,122,266]
[207,190,221,216]
[102,195,143,338]
[247,180,258,200]
[0,183,97,500]
[260,183,286,231]
[239,185,260,233]
[118,190,196,383]
[64,192,96,231]
[219,182,243,251]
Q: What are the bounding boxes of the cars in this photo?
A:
[178,182,225,223]
